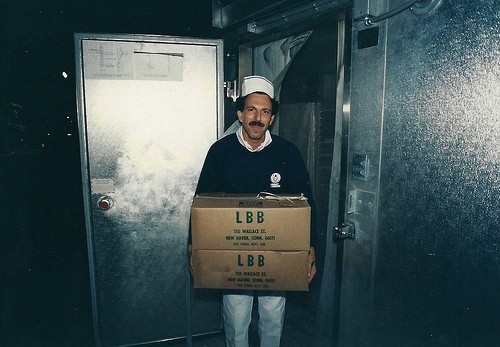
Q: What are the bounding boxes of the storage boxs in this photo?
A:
[189,195,311,251]
[190,247,309,292]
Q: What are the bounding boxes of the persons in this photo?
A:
[188,76,315,347]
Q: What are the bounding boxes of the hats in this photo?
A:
[240,74,275,100]
